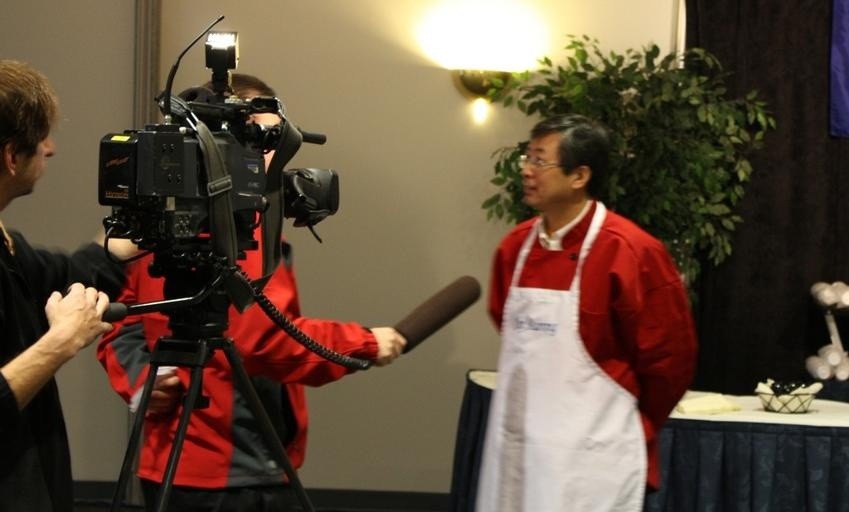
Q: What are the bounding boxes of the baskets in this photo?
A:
[754,380,818,414]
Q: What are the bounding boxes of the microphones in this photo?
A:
[394,275,481,354]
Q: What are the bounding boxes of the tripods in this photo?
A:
[110,340,316,512]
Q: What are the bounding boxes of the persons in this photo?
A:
[475,114,699,511]
[0,59,151,512]
[95,74,408,512]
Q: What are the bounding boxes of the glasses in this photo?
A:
[516,153,581,174]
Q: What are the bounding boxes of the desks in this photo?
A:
[451,369,849,512]
